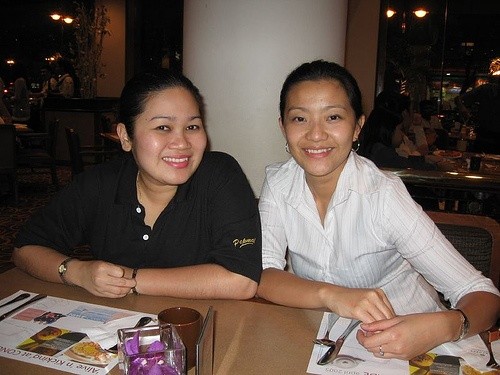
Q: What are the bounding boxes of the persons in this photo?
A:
[0,59,81,132]
[360,58,500,212]
[13,65,262,300]
[257,61,500,362]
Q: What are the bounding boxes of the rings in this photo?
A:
[379,346,383,356]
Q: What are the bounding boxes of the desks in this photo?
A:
[31,96,122,170]
[99,132,120,162]
[0,266,332,375]
[380,167,500,213]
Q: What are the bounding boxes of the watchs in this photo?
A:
[448,308,470,343]
[58,257,76,284]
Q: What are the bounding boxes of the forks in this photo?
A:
[478,329,500,370]
[104,317,151,354]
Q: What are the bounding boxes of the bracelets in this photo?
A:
[131,268,141,295]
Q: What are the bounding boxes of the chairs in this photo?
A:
[17,117,61,204]
[66,126,120,172]
[424,210,500,291]
[0,123,16,204]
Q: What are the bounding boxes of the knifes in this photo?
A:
[317,317,361,366]
[0,293,47,322]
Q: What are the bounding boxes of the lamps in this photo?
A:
[386,10,427,17]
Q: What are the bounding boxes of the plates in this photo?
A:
[63,340,117,366]
[433,148,500,171]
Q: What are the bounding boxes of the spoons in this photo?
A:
[313,313,339,345]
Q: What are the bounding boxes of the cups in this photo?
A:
[461,152,472,169]
[469,155,482,170]
[158,307,203,371]
[118,321,186,375]
[451,123,474,137]
